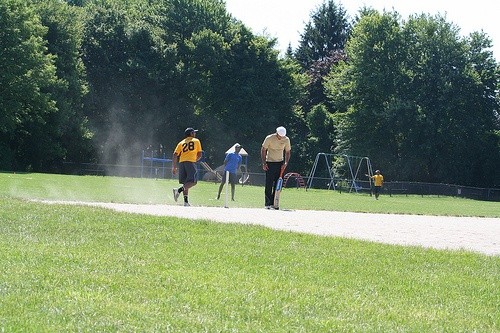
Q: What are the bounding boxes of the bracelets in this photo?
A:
[263,163,266,165]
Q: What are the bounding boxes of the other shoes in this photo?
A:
[265,205,271,209]
[184,202,191,206]
[173,188,181,202]
[271,205,274,208]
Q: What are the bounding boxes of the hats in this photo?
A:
[235,144,243,149]
[276,126,287,137]
[183,128,199,135]
[375,170,380,173]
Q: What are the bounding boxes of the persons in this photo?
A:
[215,145,242,201]
[365,170,383,200]
[173,128,202,206]
[261,126,291,209]
